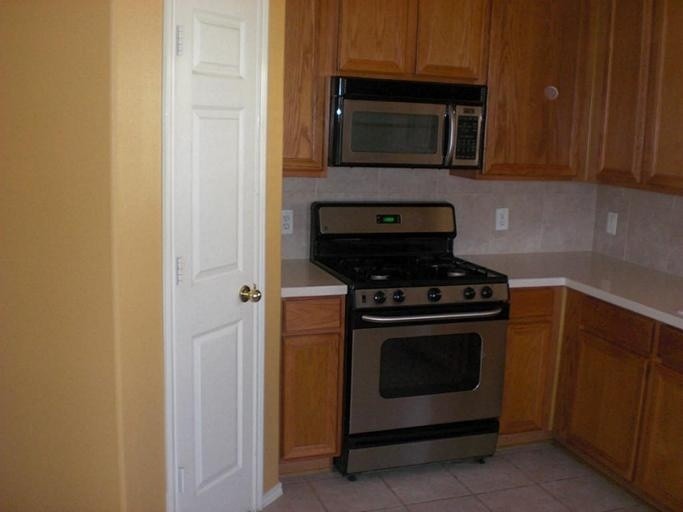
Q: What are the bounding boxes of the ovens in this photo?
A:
[346,313,503,473]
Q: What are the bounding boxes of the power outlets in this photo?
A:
[606,211,618,236]
[281,210,294,235]
[495,208,509,231]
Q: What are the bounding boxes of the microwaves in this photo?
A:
[331,75,483,169]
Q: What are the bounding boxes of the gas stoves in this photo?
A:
[314,205,514,307]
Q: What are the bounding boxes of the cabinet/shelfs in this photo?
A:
[457,4,600,182]
[552,289,683,512]
[596,5,681,197]
[338,2,486,84]
[280,295,345,463]
[284,3,340,179]
[497,286,562,434]
